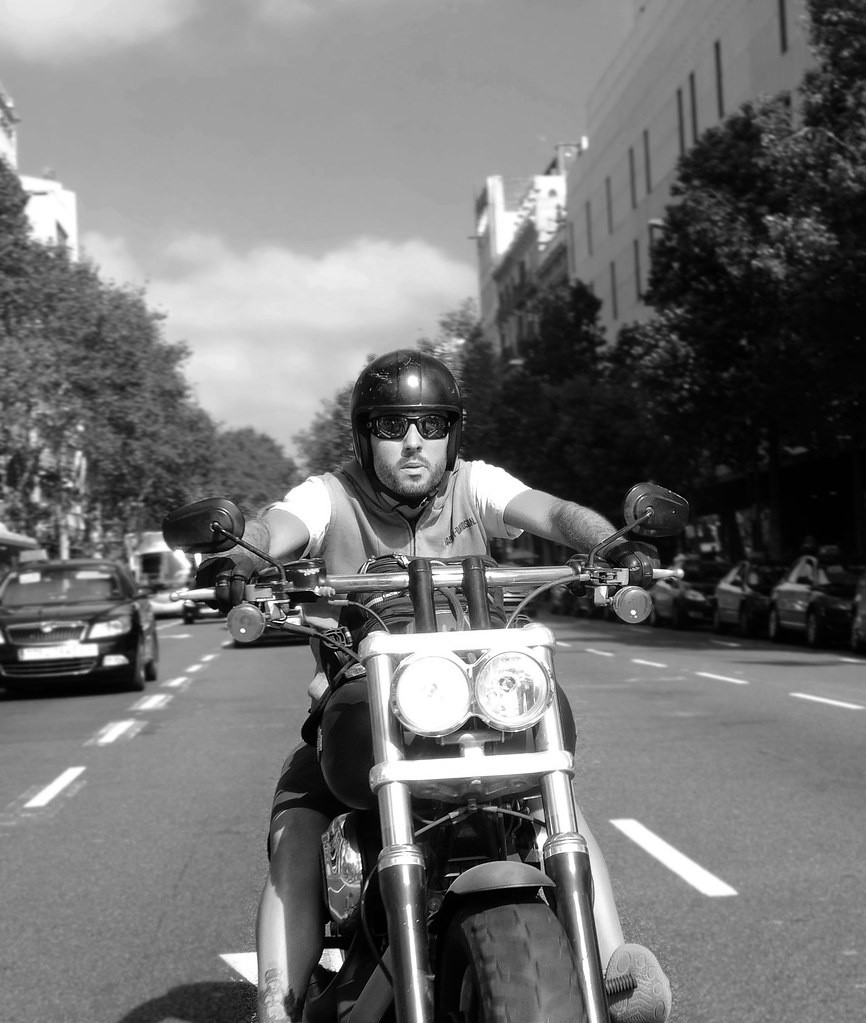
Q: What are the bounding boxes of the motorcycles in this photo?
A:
[156,481,696,1023]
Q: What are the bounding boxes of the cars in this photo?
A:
[116,491,866,656]
[1,558,160,692]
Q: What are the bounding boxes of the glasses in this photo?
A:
[369,413,451,440]
[603,541,663,589]
[198,553,258,611]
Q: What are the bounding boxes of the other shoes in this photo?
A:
[603,942,672,1021]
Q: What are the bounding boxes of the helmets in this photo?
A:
[351,350,462,480]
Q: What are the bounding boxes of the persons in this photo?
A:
[194,346,672,1023]
